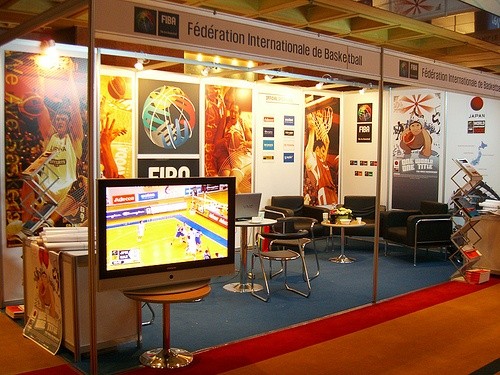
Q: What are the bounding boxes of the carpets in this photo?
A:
[0,226,460,375]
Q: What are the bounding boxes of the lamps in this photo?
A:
[46,40,57,54]
[134,57,150,70]
[359,83,372,95]
[264,68,282,81]
[316,74,332,90]
[201,63,215,76]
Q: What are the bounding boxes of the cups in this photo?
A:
[356,217,362,224]
[259,212,265,221]
[330,214,338,223]
[323,213,328,223]
[251,217,262,224]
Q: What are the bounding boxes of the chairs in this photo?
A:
[344,196,453,267]
[251,196,330,301]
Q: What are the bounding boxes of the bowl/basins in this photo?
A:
[340,219,351,225]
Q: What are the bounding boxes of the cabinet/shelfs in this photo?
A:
[449,168,483,283]
[22,237,143,362]
[463,217,500,274]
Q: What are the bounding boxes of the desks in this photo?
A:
[122,285,212,369]
[222,218,277,293]
[321,220,366,264]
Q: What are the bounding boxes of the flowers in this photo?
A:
[330,204,353,217]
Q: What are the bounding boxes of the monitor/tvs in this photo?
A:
[235,193,261,221]
[96,176,236,295]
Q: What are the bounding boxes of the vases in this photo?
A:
[335,216,348,224]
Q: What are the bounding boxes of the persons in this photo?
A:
[22,62,251,316]
[306,112,338,220]
